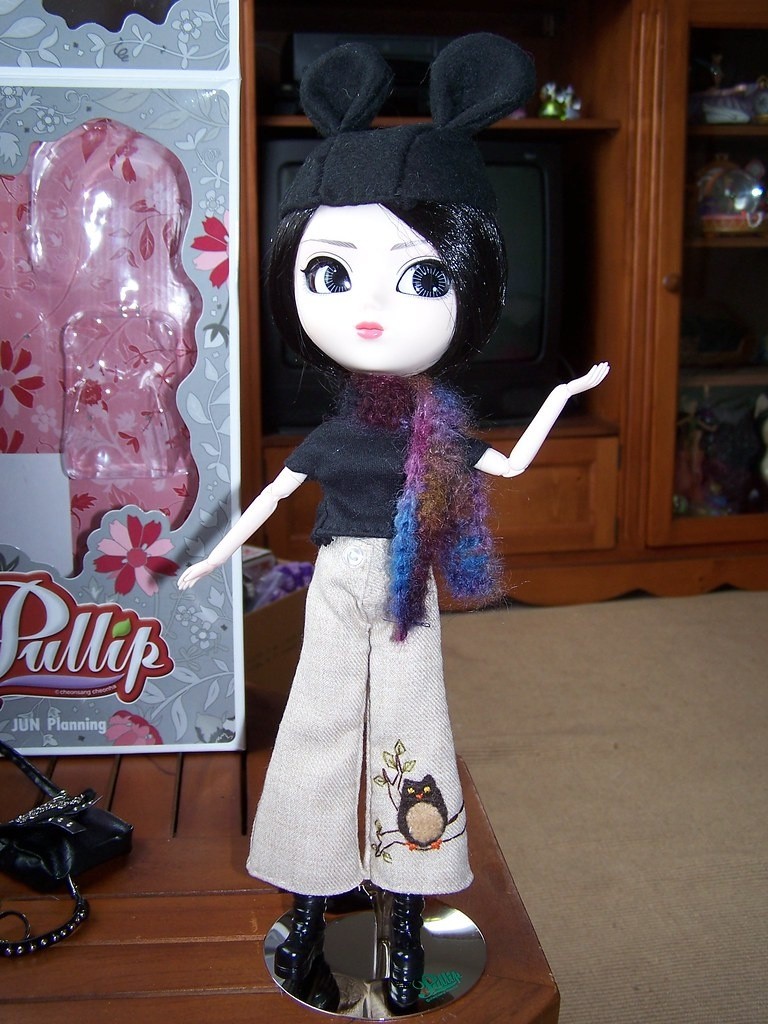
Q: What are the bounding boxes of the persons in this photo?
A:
[177,32,610,1006]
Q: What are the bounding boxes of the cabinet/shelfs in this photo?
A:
[238,0,767,649]
